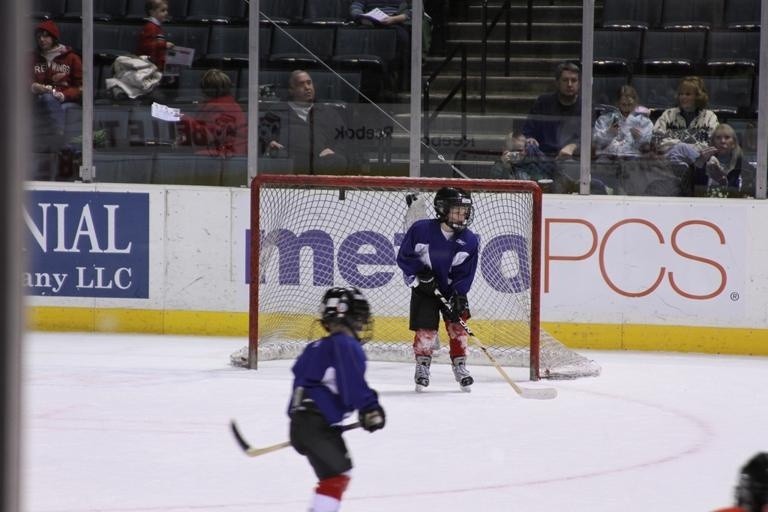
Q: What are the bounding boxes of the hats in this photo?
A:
[34,20,60,41]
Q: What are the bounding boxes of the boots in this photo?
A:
[451,355,474,387]
[415,356,431,386]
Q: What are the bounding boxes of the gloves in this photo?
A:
[415,269,442,298]
[358,406,385,433]
[441,294,466,321]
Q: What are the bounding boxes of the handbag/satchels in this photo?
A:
[486,145,580,193]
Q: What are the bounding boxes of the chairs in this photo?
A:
[580,2,757,194]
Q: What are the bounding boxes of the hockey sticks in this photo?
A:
[228,419,360,455]
[434,287,558,399]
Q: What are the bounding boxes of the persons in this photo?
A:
[141,2,180,102]
[679,123,756,199]
[288,288,385,512]
[489,132,541,193]
[351,1,434,101]
[395,186,478,394]
[170,69,249,159]
[524,63,613,196]
[258,70,348,176]
[30,21,82,145]
[652,77,718,167]
[710,453,768,511]
[592,84,654,183]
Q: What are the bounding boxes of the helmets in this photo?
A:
[320,286,375,346]
[434,187,474,232]
